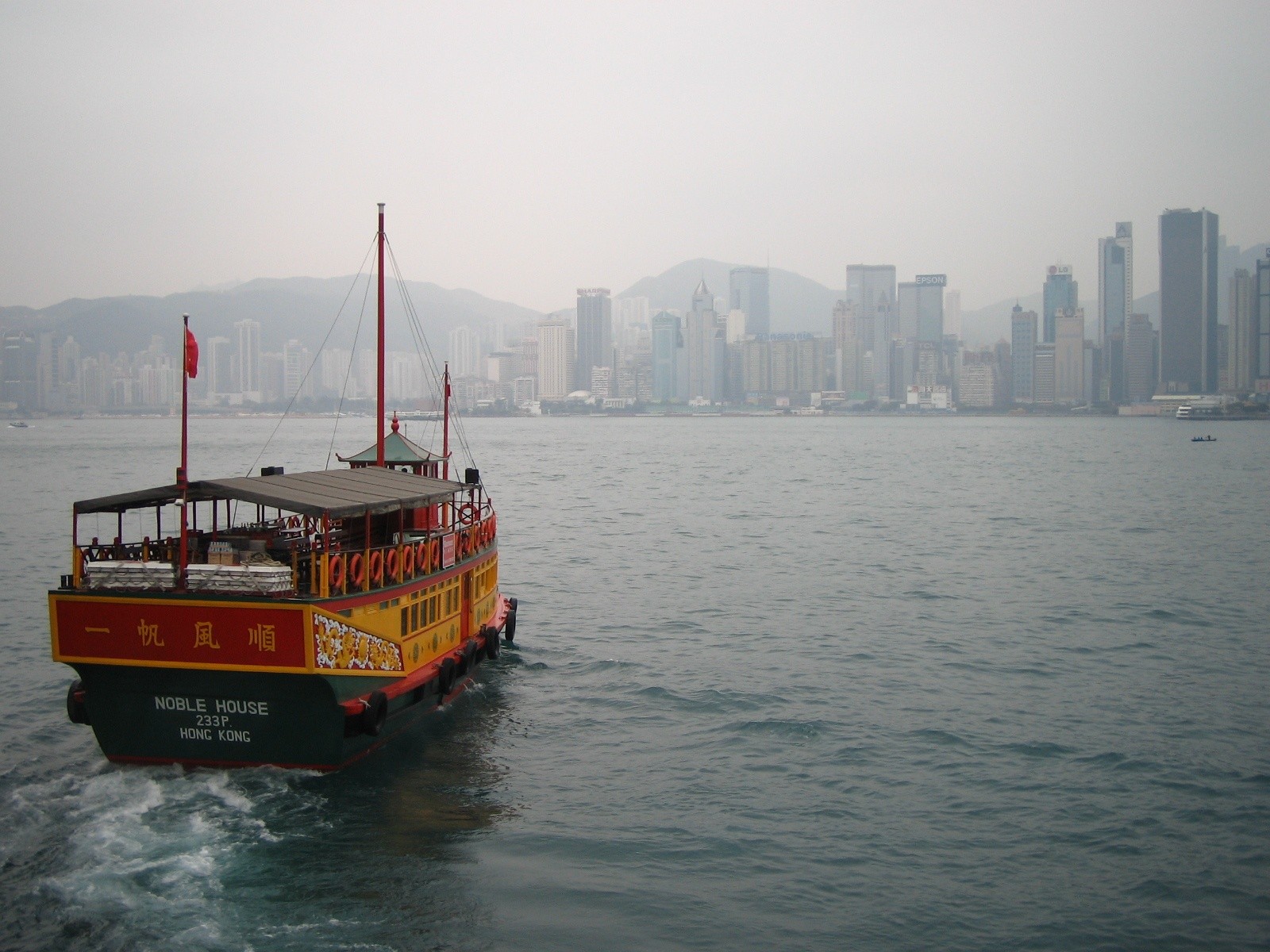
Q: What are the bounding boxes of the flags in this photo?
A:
[183,328,199,378]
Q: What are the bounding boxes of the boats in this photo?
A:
[45,201,520,775]
[1191,434,1218,441]
[9,421,28,427]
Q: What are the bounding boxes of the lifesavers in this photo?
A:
[105,548,116,559]
[487,520,493,537]
[458,504,478,525]
[464,530,471,555]
[403,546,413,575]
[474,526,480,547]
[431,540,440,568]
[80,550,94,577]
[369,552,382,584]
[349,553,365,586]
[417,543,427,571]
[290,517,300,534]
[328,556,346,590]
[455,533,461,557]
[492,514,496,534]
[386,549,399,579]
[305,514,318,534]
[481,522,488,541]
